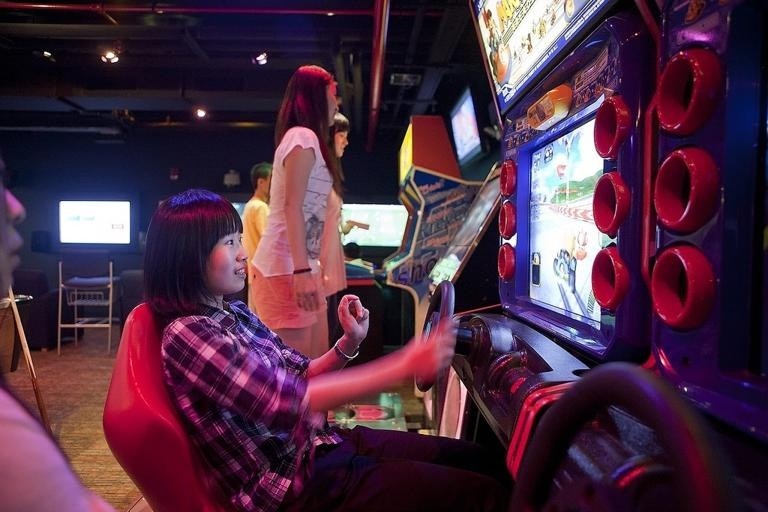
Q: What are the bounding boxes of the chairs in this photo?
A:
[58,250,120,355]
[11,270,83,351]
[328,279,390,360]
[102,303,226,512]
[121,268,144,330]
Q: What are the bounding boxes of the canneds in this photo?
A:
[380,393,402,418]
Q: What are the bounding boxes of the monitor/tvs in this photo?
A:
[432,177,505,271]
[338,197,410,254]
[527,105,607,337]
[215,192,250,216]
[49,191,138,251]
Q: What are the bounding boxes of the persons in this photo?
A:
[317,110,357,423]
[0,145,130,510]
[343,242,361,258]
[239,160,273,306]
[142,189,515,510]
[251,66,342,362]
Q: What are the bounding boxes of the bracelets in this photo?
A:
[334,335,360,362]
[293,267,312,275]
